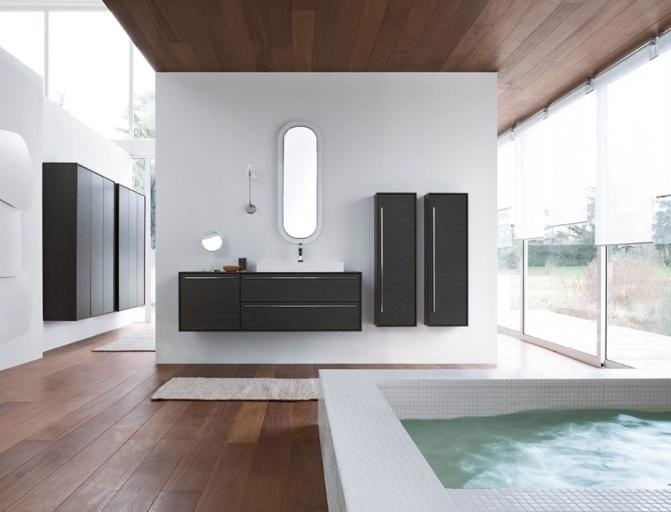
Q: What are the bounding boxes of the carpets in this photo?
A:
[91,328,155,353]
[150,373,319,404]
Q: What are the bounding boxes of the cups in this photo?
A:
[239,257,247,272]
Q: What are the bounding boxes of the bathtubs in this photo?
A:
[314,363,671,512]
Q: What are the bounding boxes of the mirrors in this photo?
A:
[198,229,225,273]
[276,120,323,247]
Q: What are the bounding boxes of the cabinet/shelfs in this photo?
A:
[424,191,473,328]
[241,271,363,333]
[373,190,420,328]
[176,269,242,334]
[114,182,150,312]
[40,160,118,323]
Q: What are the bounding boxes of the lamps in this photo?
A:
[245,161,258,214]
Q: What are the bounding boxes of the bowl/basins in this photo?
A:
[223,265,240,272]
